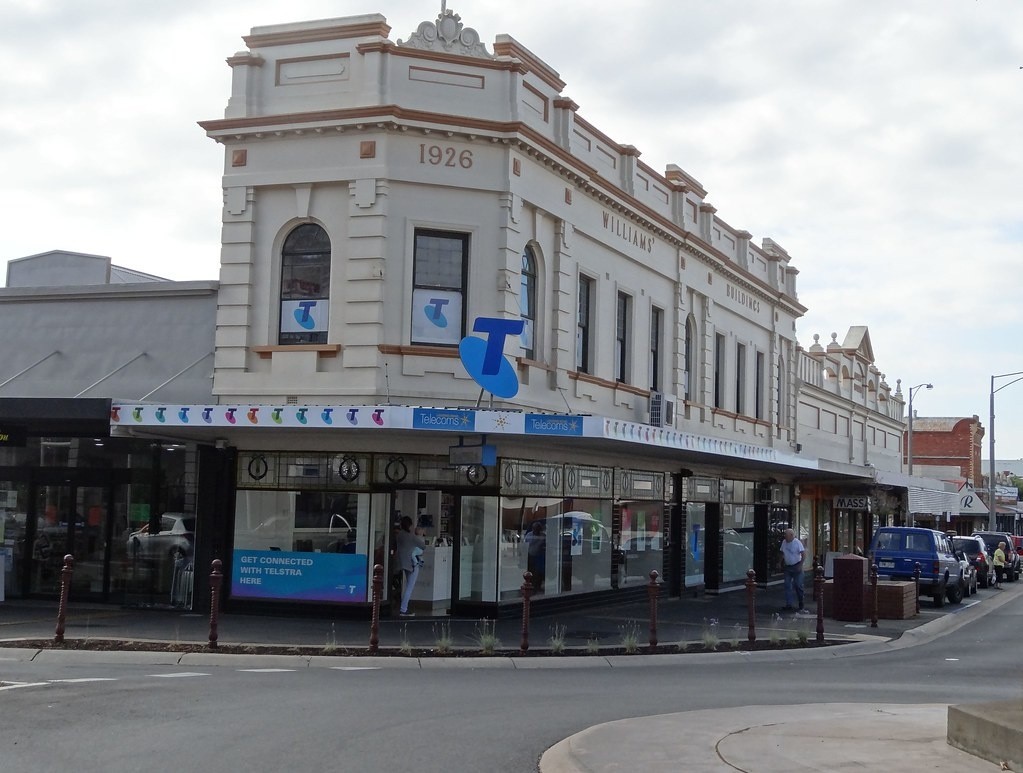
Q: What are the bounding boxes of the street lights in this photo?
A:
[908,383,934,475]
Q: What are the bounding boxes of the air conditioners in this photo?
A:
[649,391,676,430]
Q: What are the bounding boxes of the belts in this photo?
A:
[785,561,802,567]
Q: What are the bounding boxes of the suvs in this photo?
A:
[871,526,964,607]
[971,530,1020,582]
[947,534,996,589]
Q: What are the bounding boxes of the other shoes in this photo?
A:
[782,606,792,610]
[799,602,804,611]
[400,610,416,617]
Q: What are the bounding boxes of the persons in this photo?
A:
[525,522,546,590]
[394,516,426,617]
[412,526,424,567]
[780,529,806,611]
[993,542,1008,590]
[343,529,356,553]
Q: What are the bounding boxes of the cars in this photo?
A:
[518,511,610,585]
[955,551,978,597]
[838,530,865,551]
[126,512,195,568]
[621,536,693,583]
[688,527,751,577]
[5,512,47,540]
[777,522,809,549]
